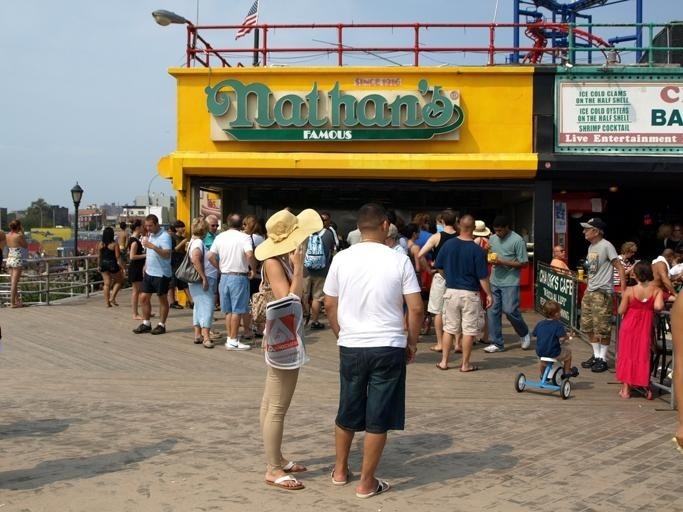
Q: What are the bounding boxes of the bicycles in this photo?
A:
[420,265,438,335]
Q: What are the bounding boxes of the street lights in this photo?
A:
[32,203,43,228]
[70,183,83,271]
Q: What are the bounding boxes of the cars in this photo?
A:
[22,231,124,271]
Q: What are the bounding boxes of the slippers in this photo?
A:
[266,475,305,490]
[356,478,390,498]
[430,344,443,352]
[283,461,306,472]
[619,388,631,398]
[436,362,447,370]
[331,464,349,484]
[646,384,653,400]
[460,364,479,371]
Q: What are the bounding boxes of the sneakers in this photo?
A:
[195,334,215,348]
[170,302,183,309]
[483,344,502,353]
[225,340,251,351]
[520,332,531,349]
[133,323,152,334]
[151,325,166,334]
[581,355,608,372]
[309,320,324,329]
[564,367,578,378]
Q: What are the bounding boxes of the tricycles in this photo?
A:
[512,330,580,401]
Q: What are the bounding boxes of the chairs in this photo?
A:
[650,312,672,395]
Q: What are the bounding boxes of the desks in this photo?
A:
[578,278,620,325]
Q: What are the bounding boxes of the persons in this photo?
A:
[532,300,578,381]
[323,202,425,499]
[4,220,28,308]
[668,287,683,451]
[612,222,683,316]
[248,207,323,491]
[386,210,531,373]
[616,261,664,400]
[301,213,363,328]
[580,217,626,372]
[97,213,266,350]
[549,245,571,275]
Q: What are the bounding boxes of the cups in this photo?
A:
[143,236,147,242]
[577,269,583,279]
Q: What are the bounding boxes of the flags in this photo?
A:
[234,1,258,40]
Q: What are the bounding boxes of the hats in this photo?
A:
[253,209,323,261]
[580,218,606,230]
[473,220,490,237]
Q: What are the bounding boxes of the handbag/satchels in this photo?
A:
[252,291,277,323]
[175,254,204,283]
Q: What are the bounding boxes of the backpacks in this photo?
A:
[304,227,327,270]
